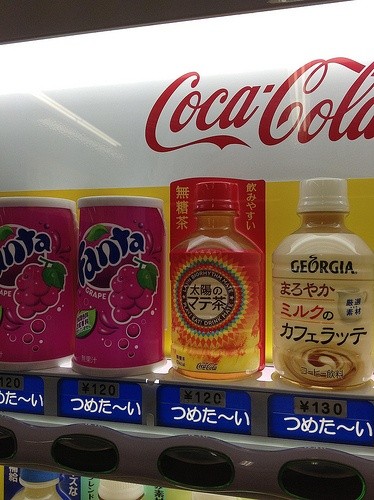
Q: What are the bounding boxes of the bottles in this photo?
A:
[272,177,373,390]
[10,468,68,500]
[167,180,267,382]
